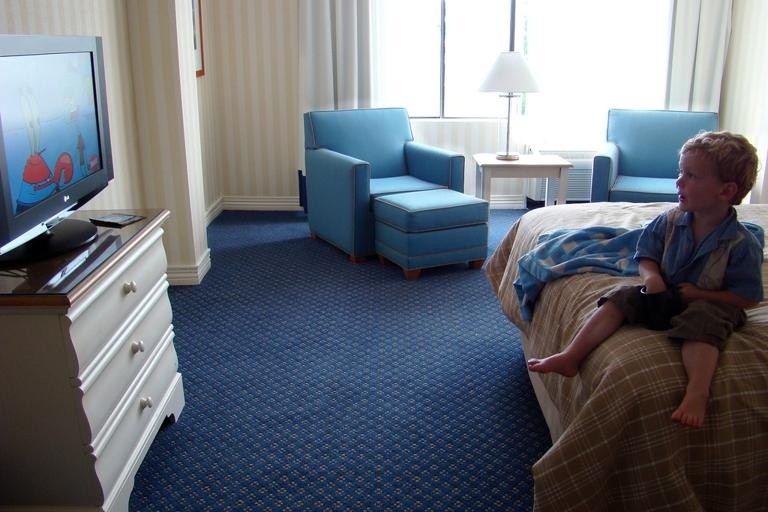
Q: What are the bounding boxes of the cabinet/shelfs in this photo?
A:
[0,210,185,512]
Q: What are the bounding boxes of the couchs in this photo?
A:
[303,107,465,263]
[591,109,718,202]
[374,189,488,280]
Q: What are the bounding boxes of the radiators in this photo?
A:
[526,151,593,209]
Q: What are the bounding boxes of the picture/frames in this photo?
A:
[193,0,204,76]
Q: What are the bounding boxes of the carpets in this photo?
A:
[129,205,553,512]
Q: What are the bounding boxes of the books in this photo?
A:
[90,213,147,227]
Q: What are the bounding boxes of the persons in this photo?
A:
[527,130,765,427]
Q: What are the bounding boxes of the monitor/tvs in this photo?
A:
[0,33,115,267]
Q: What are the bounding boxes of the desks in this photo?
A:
[473,154,575,207]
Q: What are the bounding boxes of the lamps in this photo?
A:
[479,52,539,160]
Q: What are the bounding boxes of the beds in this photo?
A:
[485,201,768,512]
